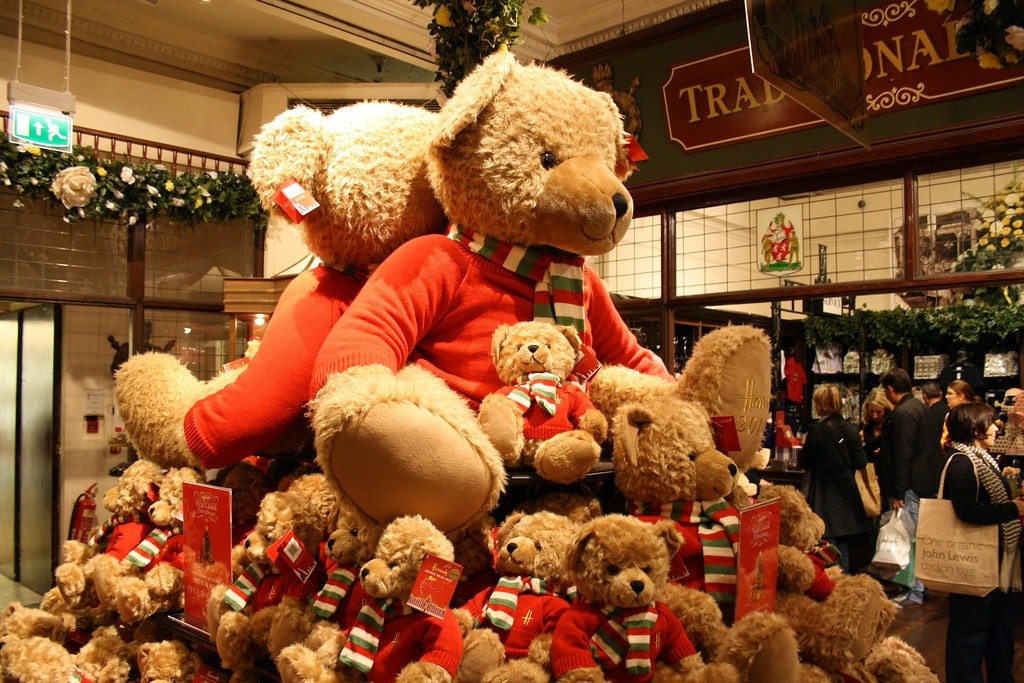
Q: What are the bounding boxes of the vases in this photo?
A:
[1007,251,1024,307]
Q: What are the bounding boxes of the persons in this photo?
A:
[797,366,981,607]
[933,402,1024,683]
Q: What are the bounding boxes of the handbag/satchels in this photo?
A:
[914,453,999,598]
[869,509,916,587]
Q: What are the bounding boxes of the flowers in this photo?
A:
[408,0,548,99]
[950,181,1024,308]
[923,0,1024,69]
[0,130,266,232]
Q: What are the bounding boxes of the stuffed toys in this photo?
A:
[112,96,454,475]
[477,319,609,486]
[305,52,776,538]
[0,390,940,682]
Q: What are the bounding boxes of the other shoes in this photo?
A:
[888,594,921,609]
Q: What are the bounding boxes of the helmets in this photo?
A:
[1000,388,1024,411]
